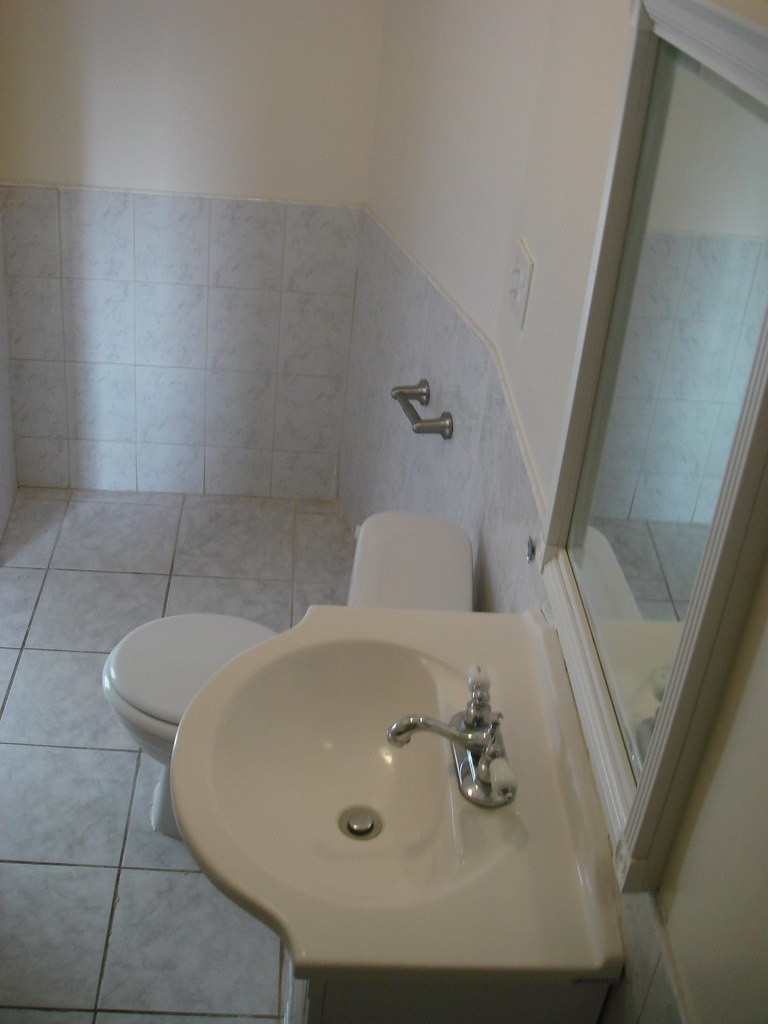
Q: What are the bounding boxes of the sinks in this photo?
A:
[211,633,506,917]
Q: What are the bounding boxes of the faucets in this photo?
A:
[387,713,501,765]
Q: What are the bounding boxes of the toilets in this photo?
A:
[99,511,474,841]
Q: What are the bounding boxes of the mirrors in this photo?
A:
[533,0,768,897]
